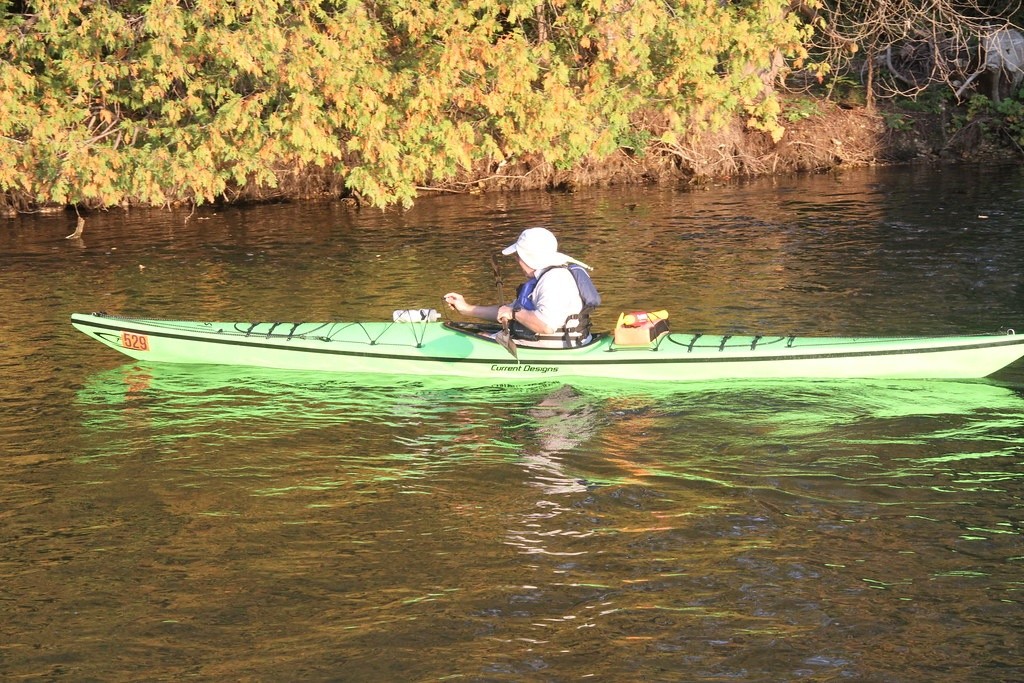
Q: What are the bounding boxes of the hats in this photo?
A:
[502,227,569,271]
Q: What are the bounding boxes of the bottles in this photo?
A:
[393,309,441,323]
[624,311,647,327]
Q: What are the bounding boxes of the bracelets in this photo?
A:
[512,306,518,321]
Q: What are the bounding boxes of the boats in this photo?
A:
[69,312,1023,384]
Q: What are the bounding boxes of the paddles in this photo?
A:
[492,253,519,360]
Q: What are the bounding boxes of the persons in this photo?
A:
[443,227,593,349]
